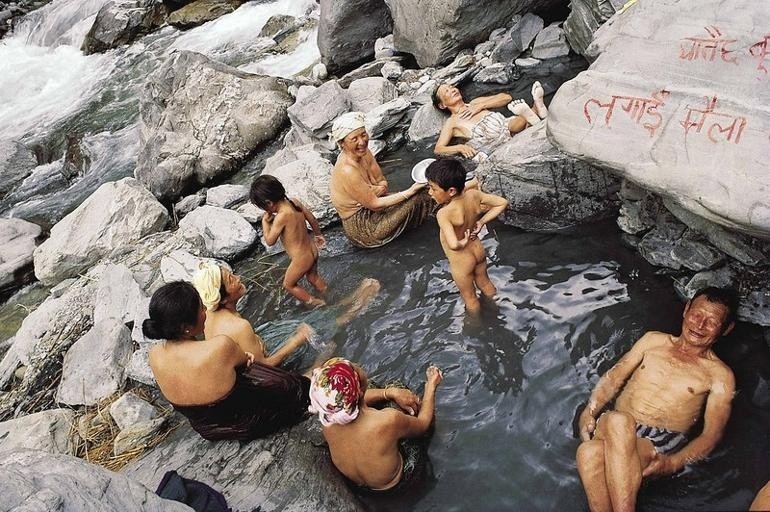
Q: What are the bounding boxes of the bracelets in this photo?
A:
[398,190,410,201]
[381,383,398,401]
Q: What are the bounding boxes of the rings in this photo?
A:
[417,396,421,402]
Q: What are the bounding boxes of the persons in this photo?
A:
[430,79,551,159]
[193,266,381,370]
[140,278,340,444]
[247,176,328,310]
[422,158,509,325]
[325,109,490,250]
[303,354,445,499]
[572,285,738,510]
